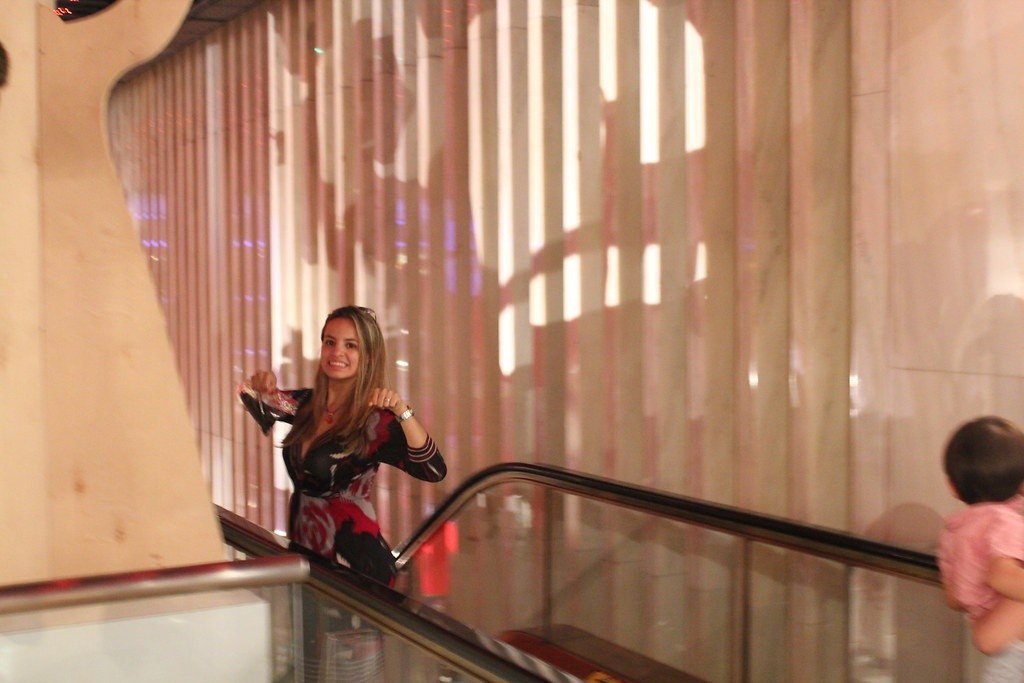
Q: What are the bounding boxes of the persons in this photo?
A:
[938,415,1024,683]
[239,305,448,683]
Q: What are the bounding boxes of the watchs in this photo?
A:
[395,405,415,422]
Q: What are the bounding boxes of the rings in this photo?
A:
[385,398,390,402]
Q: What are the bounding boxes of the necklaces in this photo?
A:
[326,399,341,424]
